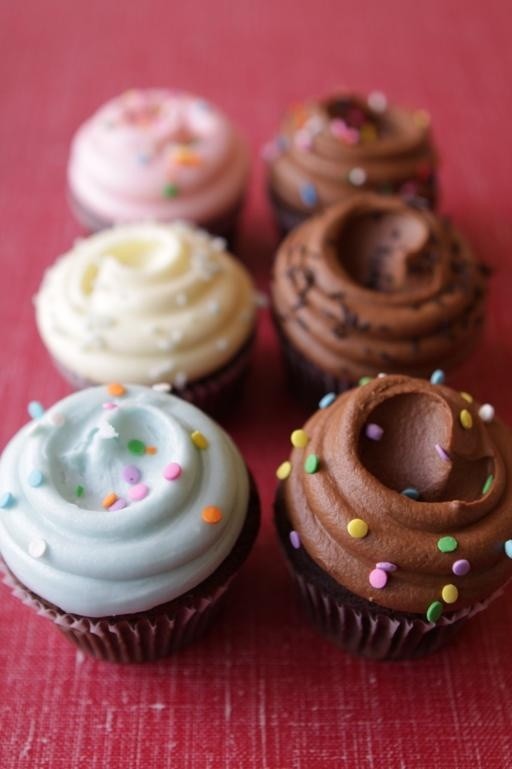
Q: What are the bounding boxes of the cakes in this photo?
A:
[260,87,439,246]
[32,220,259,424]
[272,369,512,661]
[0,382,261,663]
[268,191,496,393]
[67,86,254,257]
